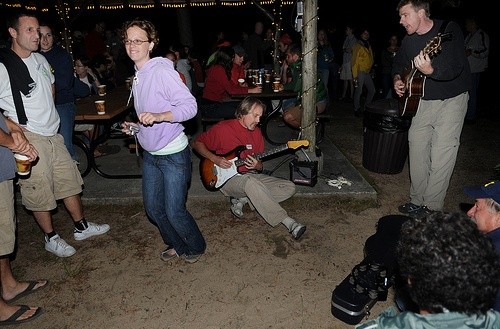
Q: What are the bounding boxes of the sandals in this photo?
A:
[230,197,244,218]
[289,220,305,239]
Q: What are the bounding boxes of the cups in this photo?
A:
[14,154,32,176]
[97,83,107,96]
[247,68,259,87]
[273,81,280,93]
[274,75,282,81]
[264,70,270,84]
[95,100,106,115]
[238,79,245,86]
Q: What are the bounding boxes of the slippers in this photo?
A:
[0,304,44,326]
[5,280,49,303]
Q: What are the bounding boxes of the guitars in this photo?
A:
[399,33,455,117]
[200,138,308,192]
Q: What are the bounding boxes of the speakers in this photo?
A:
[290,158,318,187]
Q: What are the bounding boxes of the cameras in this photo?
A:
[126,125,140,137]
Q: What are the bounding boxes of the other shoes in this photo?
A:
[273,118,285,128]
[338,96,354,104]
[465,119,474,125]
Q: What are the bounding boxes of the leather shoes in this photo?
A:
[316,122,324,144]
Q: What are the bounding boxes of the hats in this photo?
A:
[463,180,500,204]
[93,54,112,64]
[233,46,248,57]
[365,215,412,262]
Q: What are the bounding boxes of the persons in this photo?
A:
[37,23,78,161]
[228,47,247,85]
[355,208,499,328]
[188,50,205,87]
[316,30,334,95]
[1,111,49,324]
[278,33,297,110]
[202,46,262,120]
[382,33,399,98]
[462,17,489,127]
[338,27,355,101]
[393,0,470,217]
[0,12,110,257]
[74,54,107,157]
[242,21,276,71]
[206,38,231,64]
[282,46,329,144]
[122,19,206,263]
[161,49,186,84]
[460,180,500,253]
[350,29,376,114]
[170,45,192,92]
[191,95,306,240]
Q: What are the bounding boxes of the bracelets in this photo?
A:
[93,73,97,82]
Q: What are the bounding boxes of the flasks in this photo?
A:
[259,72,264,85]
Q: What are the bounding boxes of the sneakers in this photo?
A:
[73,221,110,241]
[161,249,178,262]
[185,254,201,263]
[44,234,76,258]
[400,203,419,212]
[409,205,433,220]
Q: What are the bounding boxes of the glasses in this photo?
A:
[123,38,149,45]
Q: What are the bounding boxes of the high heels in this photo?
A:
[352,105,362,117]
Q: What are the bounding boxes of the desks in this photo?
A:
[232,77,297,145]
[73,86,144,178]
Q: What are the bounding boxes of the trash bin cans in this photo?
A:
[361,98,411,175]
